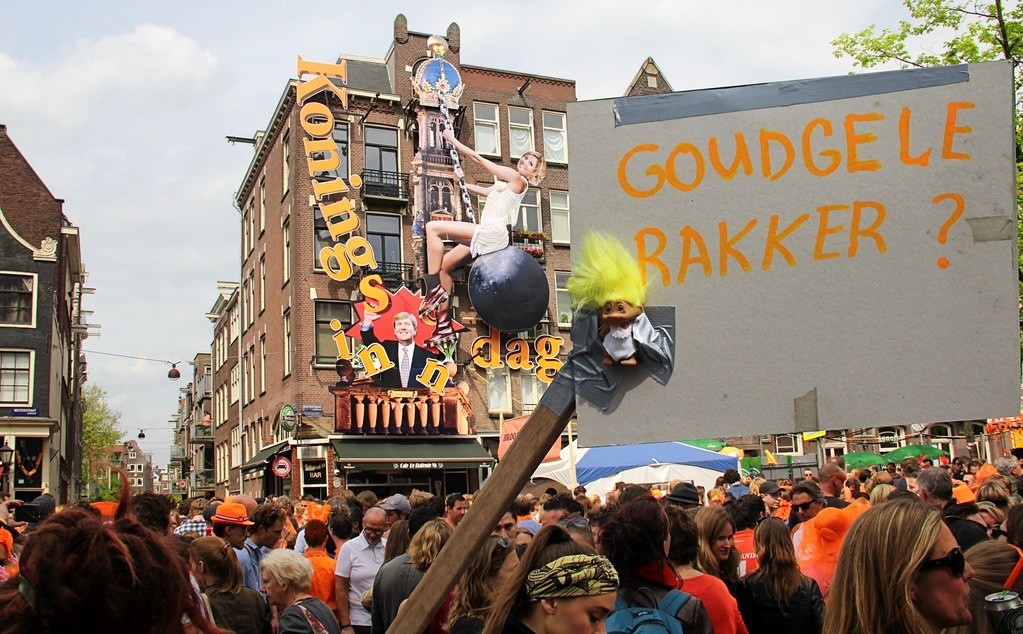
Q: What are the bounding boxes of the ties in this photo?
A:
[401,348,409,388]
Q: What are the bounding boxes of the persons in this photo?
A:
[0,455,1023,634]
[567,234,647,365]
[425,129,547,292]
[359,302,438,389]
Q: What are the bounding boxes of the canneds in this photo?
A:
[983,590,1023,634]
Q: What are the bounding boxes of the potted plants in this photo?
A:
[207,479,215,487]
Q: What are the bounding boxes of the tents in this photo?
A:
[531,439,742,505]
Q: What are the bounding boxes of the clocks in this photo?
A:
[912,423,929,432]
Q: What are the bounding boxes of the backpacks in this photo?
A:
[604,586,690,634]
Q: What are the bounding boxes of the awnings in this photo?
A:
[328,433,495,471]
[238,441,287,476]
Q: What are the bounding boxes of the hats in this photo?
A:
[666,482,703,506]
[210,503,255,526]
[377,494,410,514]
[518,520,541,536]
[31,493,56,517]
[759,482,786,494]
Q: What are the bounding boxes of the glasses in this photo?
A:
[791,500,816,512]
[913,546,966,579]
[557,518,592,532]
[955,463,961,466]
[493,535,510,553]
[991,528,1008,539]
[363,524,383,535]
[805,474,813,477]
[756,516,782,525]
[981,498,1007,507]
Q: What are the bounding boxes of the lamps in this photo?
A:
[138,430,145,440]
[0,439,16,474]
[168,364,180,381]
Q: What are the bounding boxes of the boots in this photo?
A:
[424,309,457,347]
[418,273,449,318]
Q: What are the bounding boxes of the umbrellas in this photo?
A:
[842,452,892,473]
[677,438,728,453]
[884,445,950,464]
